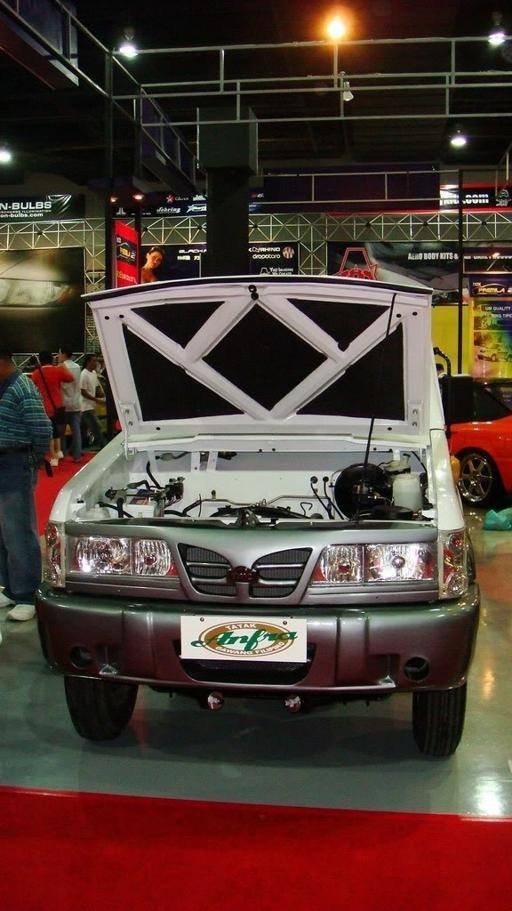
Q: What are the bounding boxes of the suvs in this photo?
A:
[34,273,481,759]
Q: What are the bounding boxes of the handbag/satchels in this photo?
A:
[54,407,66,423]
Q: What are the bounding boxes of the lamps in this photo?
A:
[487,10,507,46]
[0,142,11,162]
[342,81,355,102]
[119,27,139,57]
[450,129,468,147]
[324,15,345,39]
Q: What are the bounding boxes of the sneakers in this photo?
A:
[1,591,15,609]
[6,603,36,622]
[46,450,64,467]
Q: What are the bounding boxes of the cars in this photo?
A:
[63,373,106,447]
[479,342,508,362]
[475,304,500,329]
[186,204,207,213]
[472,283,512,297]
[439,375,512,509]
[0,247,84,322]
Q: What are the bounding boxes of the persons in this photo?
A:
[140,246,167,284]
[31,350,73,466]
[58,345,81,463]
[436,364,446,379]
[0,335,53,622]
[69,354,107,457]
